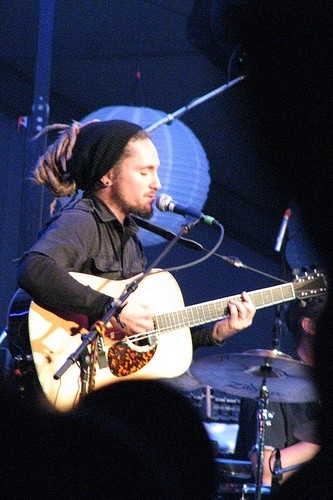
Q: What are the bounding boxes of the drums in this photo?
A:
[214,456,254,495]
[240,483,272,500]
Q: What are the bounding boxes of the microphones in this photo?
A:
[273,448,283,480]
[155,194,216,226]
[272,208,293,253]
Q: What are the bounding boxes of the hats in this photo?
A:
[67,119,143,191]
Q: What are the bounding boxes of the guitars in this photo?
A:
[6,267,329,416]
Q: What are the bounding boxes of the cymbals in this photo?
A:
[158,370,203,391]
[189,348,321,403]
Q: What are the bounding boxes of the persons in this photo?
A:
[50,378,214,500]
[235,297,333,500]
[17,119,257,348]
